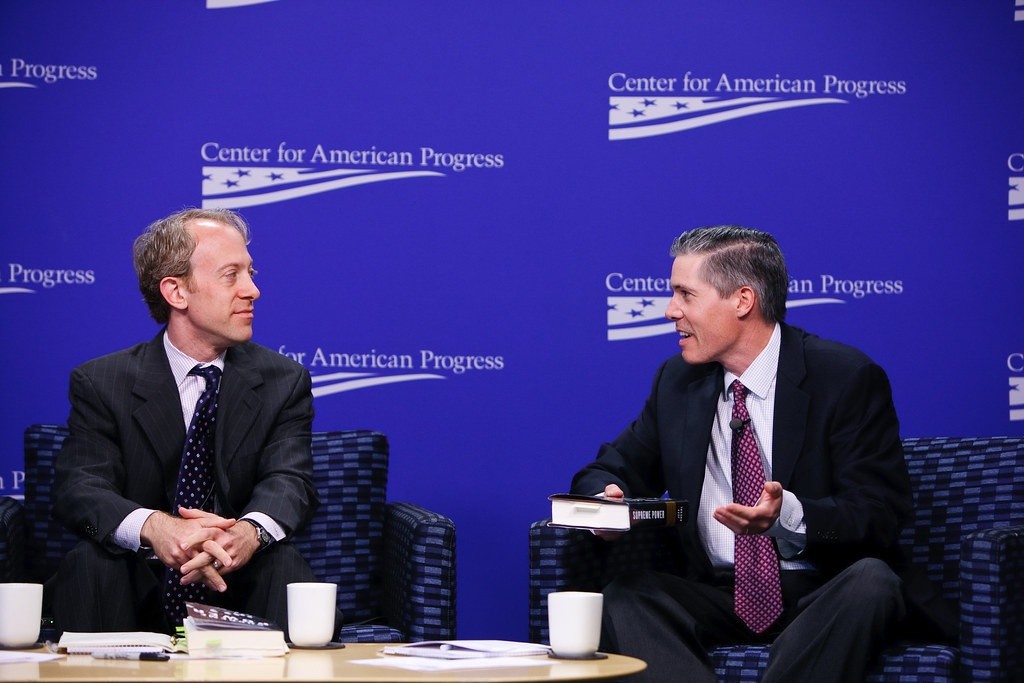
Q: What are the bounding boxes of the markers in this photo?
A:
[90,649,172,663]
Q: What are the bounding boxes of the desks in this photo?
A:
[0,644,648,683]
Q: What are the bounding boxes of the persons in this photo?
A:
[54,206,346,649]
[570,225,909,681]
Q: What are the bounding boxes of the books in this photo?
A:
[183,601,290,658]
[547,493,692,534]
[58,629,184,654]
[385,639,548,661]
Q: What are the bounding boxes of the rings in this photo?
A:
[214,562,218,568]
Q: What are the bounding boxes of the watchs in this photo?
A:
[242,518,275,547]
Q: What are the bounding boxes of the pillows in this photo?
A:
[25,422,390,625]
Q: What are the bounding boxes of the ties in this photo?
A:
[164,365,223,626]
[730,377,784,634]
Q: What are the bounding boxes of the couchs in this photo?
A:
[0,424,457,643]
[527,438,1024,683]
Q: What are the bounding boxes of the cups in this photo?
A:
[548,592,604,657]
[0,583,43,649]
[287,582,338,647]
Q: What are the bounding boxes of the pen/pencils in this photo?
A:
[440,644,459,652]
